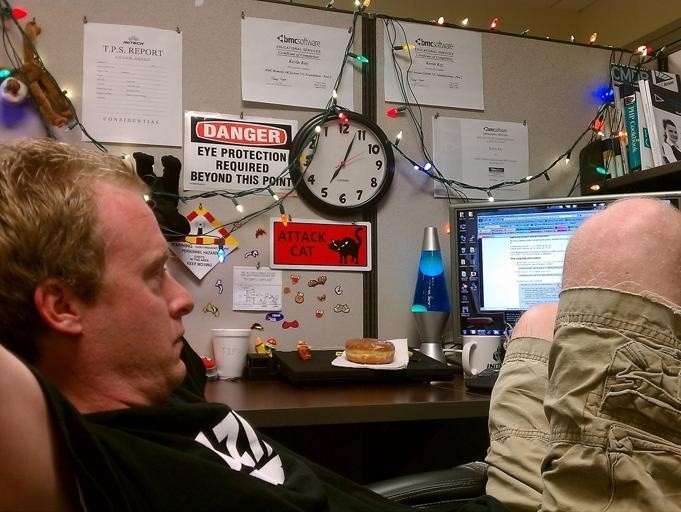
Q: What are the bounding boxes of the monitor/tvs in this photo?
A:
[448,190,681,350]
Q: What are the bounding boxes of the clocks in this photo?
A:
[289,110,395,217]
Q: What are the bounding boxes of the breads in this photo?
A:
[345,338,395,364]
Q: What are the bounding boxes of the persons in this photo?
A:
[0,136,681,512]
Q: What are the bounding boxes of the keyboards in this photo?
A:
[464,362,502,390]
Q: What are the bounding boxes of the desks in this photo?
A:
[204,377,491,429]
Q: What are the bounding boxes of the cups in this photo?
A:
[208,326,251,382]
[458,335,503,383]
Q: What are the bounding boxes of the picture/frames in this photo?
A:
[270,217,372,272]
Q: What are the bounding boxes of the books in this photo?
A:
[609,64,681,178]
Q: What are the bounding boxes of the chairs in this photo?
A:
[368,468,487,503]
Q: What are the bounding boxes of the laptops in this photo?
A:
[271,343,456,385]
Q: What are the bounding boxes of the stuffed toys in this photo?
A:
[0,21,73,129]
